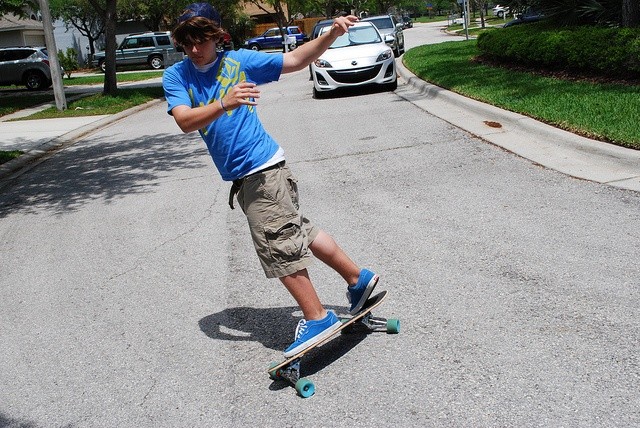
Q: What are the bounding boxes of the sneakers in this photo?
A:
[283,310,343,358]
[345,267,381,315]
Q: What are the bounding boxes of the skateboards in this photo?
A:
[267,291,400,397]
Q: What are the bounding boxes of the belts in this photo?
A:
[227,159,288,210]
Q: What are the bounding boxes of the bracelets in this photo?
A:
[220,98,229,111]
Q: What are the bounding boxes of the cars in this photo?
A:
[303,19,335,79]
[402,17,412,28]
[309,21,397,99]
[492,4,521,18]
[503,5,544,29]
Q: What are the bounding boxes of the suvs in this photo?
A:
[93,31,177,72]
[359,13,404,57]
[245,26,305,50]
[0,46,65,91]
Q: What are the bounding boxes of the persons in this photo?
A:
[160,3,381,359]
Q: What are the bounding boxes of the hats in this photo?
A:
[172,3,222,28]
